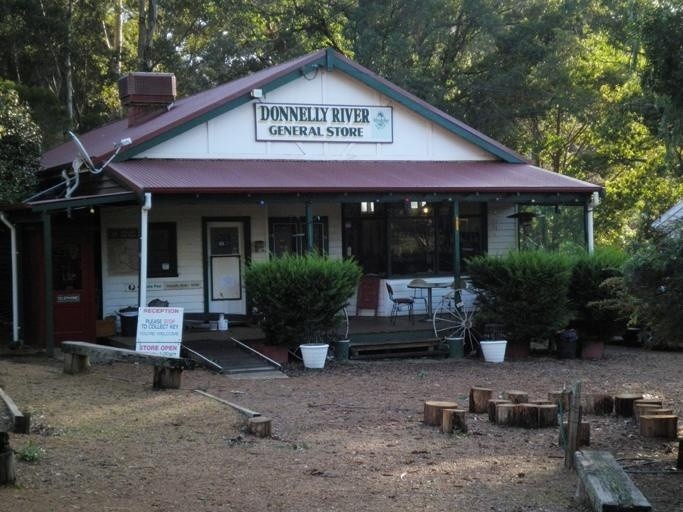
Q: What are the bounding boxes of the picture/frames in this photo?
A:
[210,255,242,301]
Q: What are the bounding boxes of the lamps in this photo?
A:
[507,205,545,223]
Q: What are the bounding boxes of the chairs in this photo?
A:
[385,282,415,326]
[439,279,466,322]
[409,280,428,317]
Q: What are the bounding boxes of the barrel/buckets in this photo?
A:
[445,336,465,359]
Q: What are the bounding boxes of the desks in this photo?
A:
[408,283,453,323]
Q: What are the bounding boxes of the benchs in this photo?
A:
[61,341,196,388]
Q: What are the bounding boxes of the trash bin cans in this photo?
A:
[116,306,138,337]
